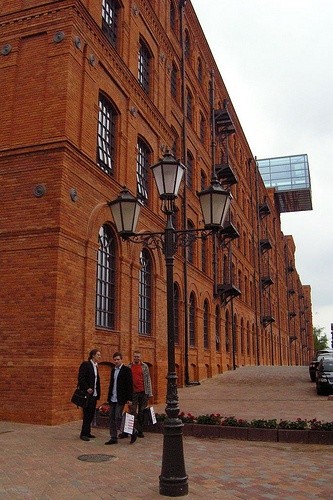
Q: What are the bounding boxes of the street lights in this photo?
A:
[104,145,235,498]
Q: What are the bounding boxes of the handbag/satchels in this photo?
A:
[120,411,135,435]
[144,406,157,425]
[71,389,89,407]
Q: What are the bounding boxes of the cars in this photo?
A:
[309,348,333,395]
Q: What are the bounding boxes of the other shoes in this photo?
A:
[80,435,90,441]
[105,438,118,445]
[130,428,138,444]
[118,433,128,438]
[137,430,144,438]
[88,434,96,438]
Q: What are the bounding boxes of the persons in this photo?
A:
[104,352,139,445]
[118,351,153,438]
[78,349,101,441]
